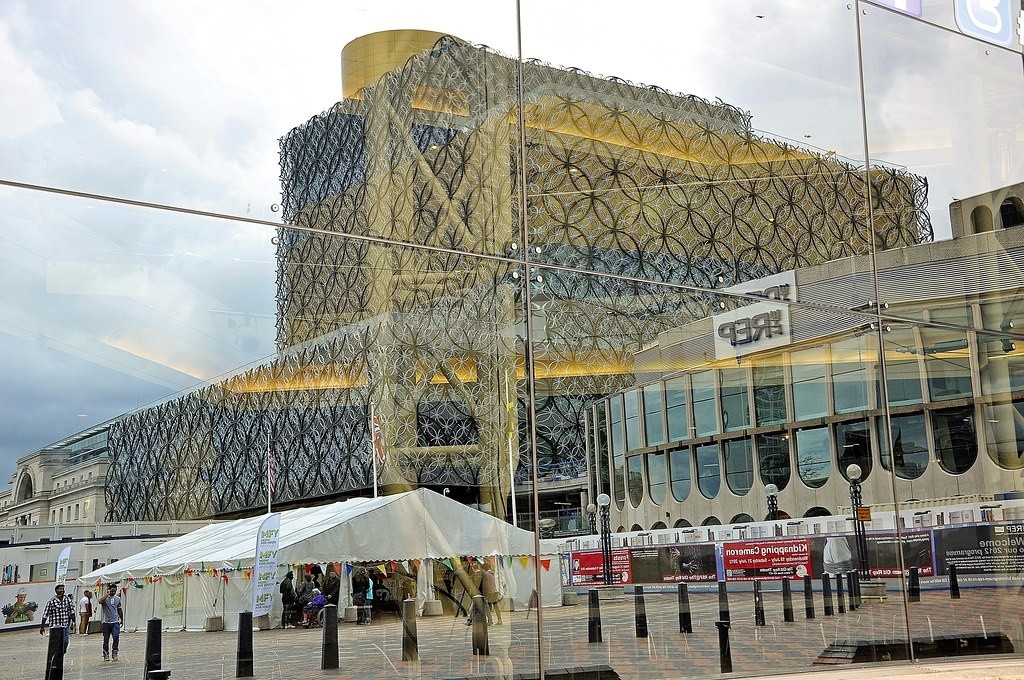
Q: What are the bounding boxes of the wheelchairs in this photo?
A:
[299,597,330,628]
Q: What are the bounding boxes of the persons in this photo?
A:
[40,584,75,680]
[63,594,76,655]
[280,571,296,629]
[462,562,504,626]
[79,590,92,637]
[295,567,389,626]
[5,587,34,624]
[98,584,123,662]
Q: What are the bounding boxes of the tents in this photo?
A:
[75,487,563,634]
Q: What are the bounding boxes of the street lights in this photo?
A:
[846,464,871,582]
[597,494,613,586]
[765,484,779,520]
[587,503,597,535]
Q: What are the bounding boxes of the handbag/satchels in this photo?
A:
[487,591,503,604]
[295,581,306,596]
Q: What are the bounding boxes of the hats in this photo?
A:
[17,587,30,595]
[285,570,293,577]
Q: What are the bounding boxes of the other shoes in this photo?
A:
[300,621,308,624]
[487,621,493,626]
[285,625,296,629]
[494,619,503,625]
[112,649,119,661]
[465,619,471,626]
[80,633,89,637]
[102,650,110,661]
